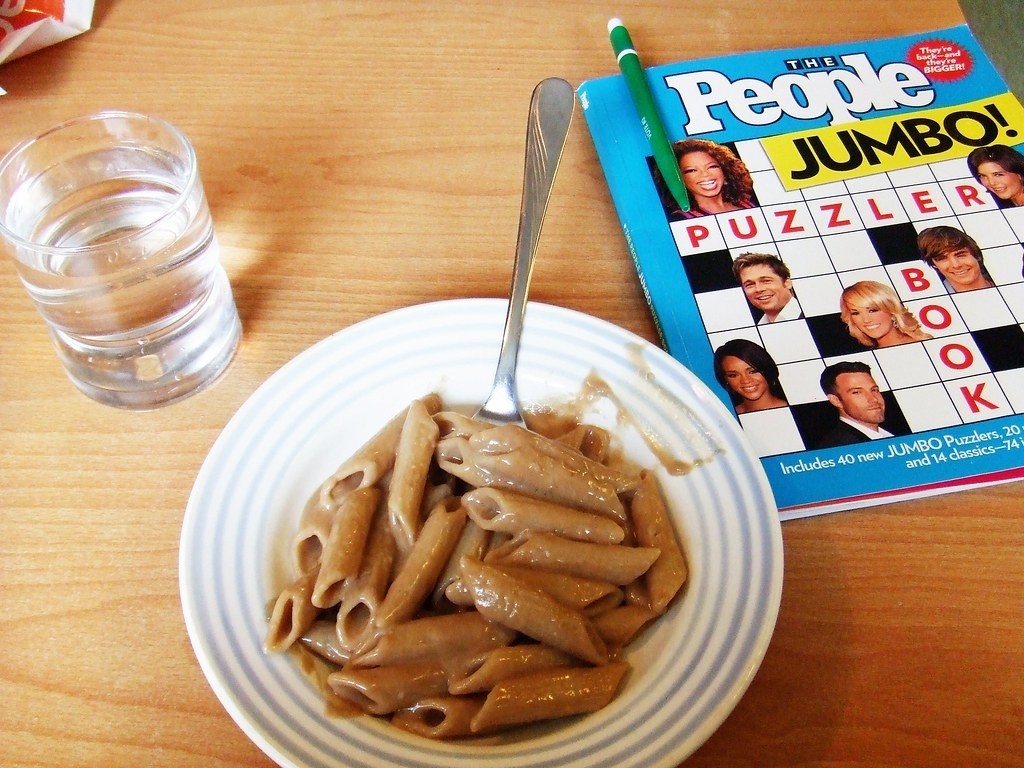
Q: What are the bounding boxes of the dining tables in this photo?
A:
[0,0,1024,767]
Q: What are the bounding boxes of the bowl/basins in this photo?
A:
[177,297,785,768]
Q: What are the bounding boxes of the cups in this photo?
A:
[0,110,242,411]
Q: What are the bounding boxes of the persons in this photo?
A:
[662,137,762,218]
[838,279,934,344]
[962,143,1022,213]
[711,337,792,412]
[913,226,990,290]
[734,252,806,324]
[817,358,905,448]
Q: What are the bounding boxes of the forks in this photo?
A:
[447,77,575,502]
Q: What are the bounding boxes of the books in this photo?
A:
[572,23,1022,523]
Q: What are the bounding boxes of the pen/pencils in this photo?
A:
[606,18,692,216]
